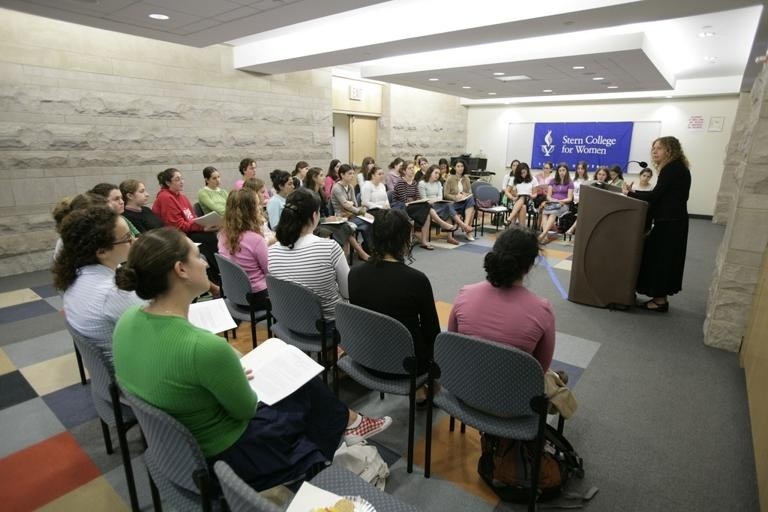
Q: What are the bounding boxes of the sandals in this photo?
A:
[540,238,551,244]
[565,226,576,235]
[502,219,511,227]
[537,235,544,243]
[639,299,669,313]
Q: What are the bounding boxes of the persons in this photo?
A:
[199,167,226,221]
[215,190,268,310]
[635,136,692,313]
[91,183,221,299]
[235,159,268,206]
[291,160,309,188]
[348,207,441,413]
[303,168,373,264]
[266,187,350,367]
[53,206,155,366]
[111,226,393,494]
[153,170,220,257]
[446,227,556,375]
[242,180,277,244]
[325,154,477,251]
[119,179,221,284]
[501,160,655,245]
[54,196,111,266]
[266,170,294,230]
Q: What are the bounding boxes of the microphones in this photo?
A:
[604,160,648,191]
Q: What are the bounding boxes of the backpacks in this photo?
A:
[479,423,585,504]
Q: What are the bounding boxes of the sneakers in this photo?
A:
[447,238,459,245]
[546,370,568,415]
[342,416,393,446]
[461,224,474,232]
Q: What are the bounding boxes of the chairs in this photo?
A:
[213,460,417,512]
[214,252,276,349]
[265,276,340,386]
[424,331,566,512]
[63,318,140,512]
[117,382,296,512]
[333,301,448,474]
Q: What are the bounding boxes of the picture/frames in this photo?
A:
[706,115,726,133]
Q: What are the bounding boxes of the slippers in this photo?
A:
[441,224,458,232]
[419,244,434,250]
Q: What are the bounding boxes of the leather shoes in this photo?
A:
[358,253,373,263]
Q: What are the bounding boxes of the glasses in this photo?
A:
[113,232,135,245]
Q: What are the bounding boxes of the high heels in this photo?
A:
[465,233,475,240]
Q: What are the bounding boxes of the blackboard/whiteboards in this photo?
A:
[505,121,662,174]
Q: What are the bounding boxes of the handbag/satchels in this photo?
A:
[331,441,390,491]
[475,198,492,208]
[543,202,561,211]
